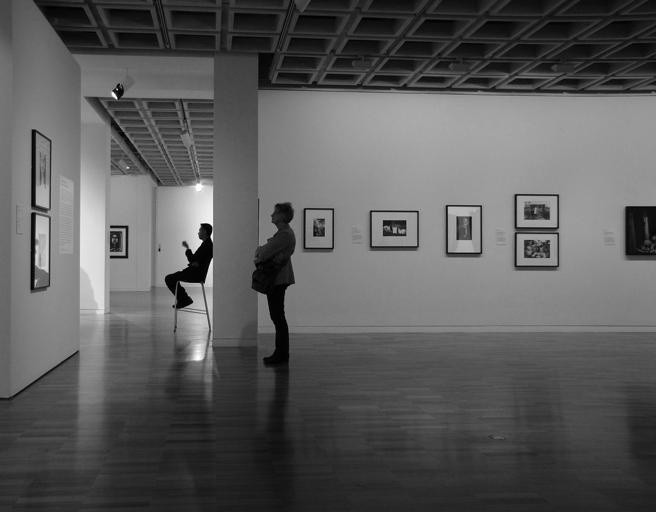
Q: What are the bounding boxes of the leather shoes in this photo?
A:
[172,297,192,308]
[267,352,288,364]
[263,351,277,361]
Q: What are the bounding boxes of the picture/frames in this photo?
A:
[514,194,559,267]
[30,129,52,290]
[304,208,334,249]
[110,226,128,258]
[446,205,482,254]
[370,210,419,248]
[625,206,656,255]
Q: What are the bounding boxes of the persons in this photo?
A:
[458,216,470,239]
[524,205,549,220]
[254,201,296,366]
[109,233,120,251]
[162,222,213,309]
[524,240,549,258]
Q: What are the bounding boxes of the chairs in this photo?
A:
[174,255,214,333]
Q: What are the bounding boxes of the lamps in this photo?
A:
[110,83,124,101]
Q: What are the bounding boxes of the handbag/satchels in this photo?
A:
[251,259,283,295]
